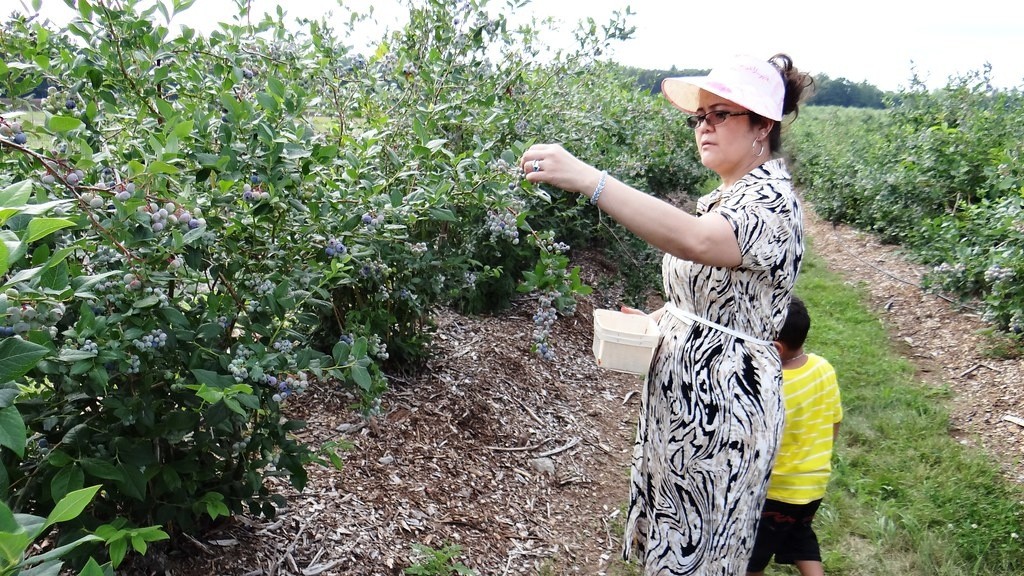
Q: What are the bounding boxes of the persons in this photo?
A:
[745,297,843,576]
[522,54,804,576]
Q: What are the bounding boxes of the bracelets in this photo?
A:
[590,169,607,205]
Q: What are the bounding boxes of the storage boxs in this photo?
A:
[592,307,659,375]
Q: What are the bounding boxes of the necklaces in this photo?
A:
[785,351,804,363]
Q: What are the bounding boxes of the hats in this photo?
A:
[661,55,785,122]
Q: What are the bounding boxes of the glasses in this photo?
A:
[686,111,750,128]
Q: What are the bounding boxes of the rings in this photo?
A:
[533,160,539,172]
